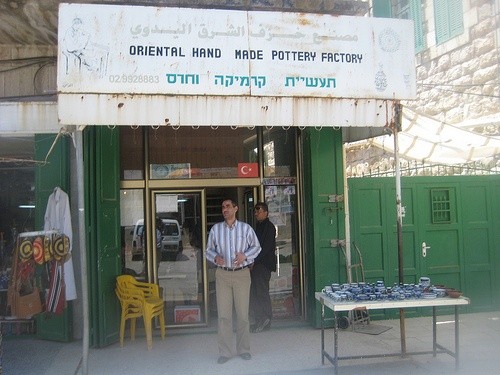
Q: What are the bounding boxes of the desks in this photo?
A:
[313,292,470,375]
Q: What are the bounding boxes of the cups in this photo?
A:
[321,280,422,303]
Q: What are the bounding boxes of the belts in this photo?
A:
[219,266,248,271]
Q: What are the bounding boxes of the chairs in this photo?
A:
[115,275,165,354]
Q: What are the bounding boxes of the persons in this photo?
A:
[203,198,262,363]
[140,210,166,281]
[251,203,277,333]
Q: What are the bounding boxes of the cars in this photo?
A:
[129,218,184,261]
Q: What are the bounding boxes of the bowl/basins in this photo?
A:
[432,284,461,298]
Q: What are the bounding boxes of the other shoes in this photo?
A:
[239,353,251,360]
[249,321,271,333]
[217,357,231,363]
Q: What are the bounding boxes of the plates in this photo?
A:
[420,293,436,300]
